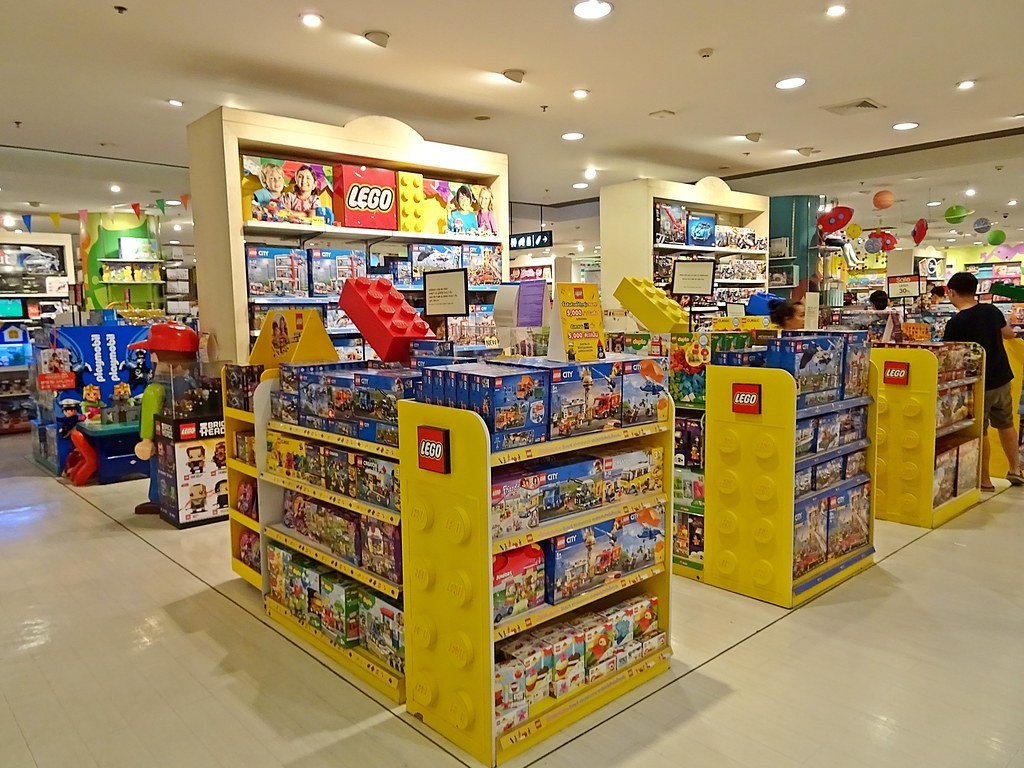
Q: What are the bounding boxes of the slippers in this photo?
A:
[976,483,996,491]
[1006,469,1024,485]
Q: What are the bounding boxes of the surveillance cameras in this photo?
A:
[1003,213,1009,219]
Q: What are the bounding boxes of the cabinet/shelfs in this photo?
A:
[0,230,81,437]
[868,341,986,529]
[221,355,674,768]
[187,106,509,379]
[599,176,770,332]
[673,361,880,609]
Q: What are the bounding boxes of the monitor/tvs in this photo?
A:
[0,297,29,320]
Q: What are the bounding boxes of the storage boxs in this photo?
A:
[0,156,1024,736]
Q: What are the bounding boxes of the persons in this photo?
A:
[58,399,86,440]
[869,290,888,310]
[284,166,321,210]
[768,300,804,329]
[254,163,284,202]
[127,321,199,514]
[929,287,945,301]
[446,186,477,231]
[477,188,497,234]
[942,272,1024,492]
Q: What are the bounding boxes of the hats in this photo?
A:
[931,286,945,298]
